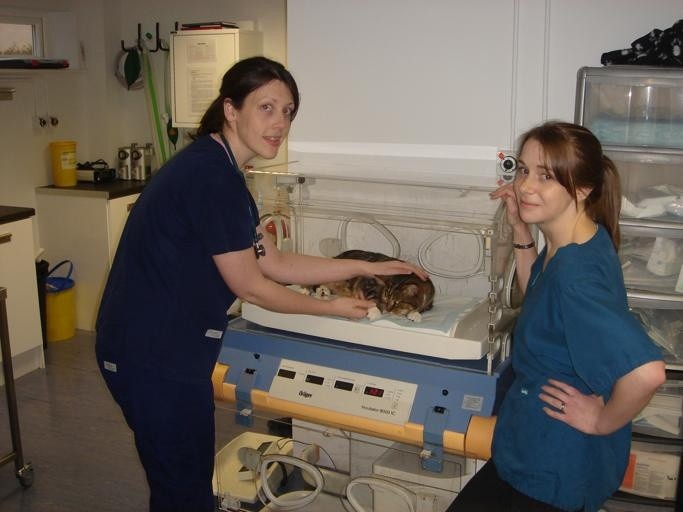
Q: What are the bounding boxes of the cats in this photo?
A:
[227,249,435,324]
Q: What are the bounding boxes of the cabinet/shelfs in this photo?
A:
[0,215,45,389]
[34,194,142,331]
[574,64,683,512]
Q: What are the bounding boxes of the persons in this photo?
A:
[95,57,430,511]
[441,122,666,512]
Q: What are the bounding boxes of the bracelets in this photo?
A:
[512,242,536,249]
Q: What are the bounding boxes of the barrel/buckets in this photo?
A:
[49,142,79,187]
[45,260,76,342]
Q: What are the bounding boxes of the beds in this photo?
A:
[237,284,513,361]
